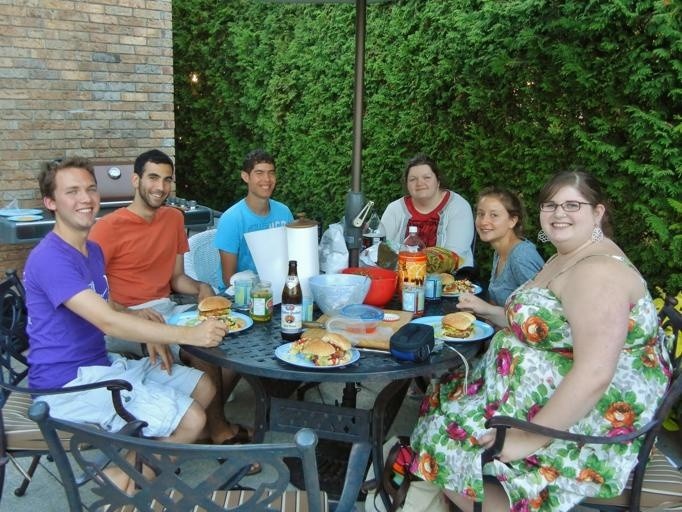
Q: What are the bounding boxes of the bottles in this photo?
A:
[280,261,303,340]
[361,208,386,251]
[398,226,427,304]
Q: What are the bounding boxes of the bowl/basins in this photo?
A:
[308,273,372,318]
[343,267,398,307]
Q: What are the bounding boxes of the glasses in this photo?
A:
[540,200,595,212]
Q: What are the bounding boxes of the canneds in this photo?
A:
[401,288,425,317]
[249,281,274,322]
[425,275,442,302]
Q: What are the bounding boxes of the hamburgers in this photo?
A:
[441,311,477,340]
[302,333,352,366]
[197,295,232,320]
[440,273,458,294]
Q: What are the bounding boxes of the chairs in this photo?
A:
[377,294,681,511]
[178,228,231,296]
[27,400,328,511]
[0,268,152,512]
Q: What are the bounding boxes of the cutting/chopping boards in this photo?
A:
[301,307,413,350]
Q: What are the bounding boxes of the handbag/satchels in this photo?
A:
[389,323,435,366]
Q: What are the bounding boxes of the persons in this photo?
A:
[24,154,227,512]
[475,188,545,307]
[86,150,262,475]
[408,171,674,512]
[210,152,295,287]
[376,158,474,267]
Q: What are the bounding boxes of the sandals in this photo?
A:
[217,437,262,476]
[226,420,254,438]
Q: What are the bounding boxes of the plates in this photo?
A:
[0,208,44,216]
[7,215,44,222]
[411,315,494,343]
[441,284,482,298]
[169,310,254,334]
[387,333,389,335]
[274,340,360,370]
